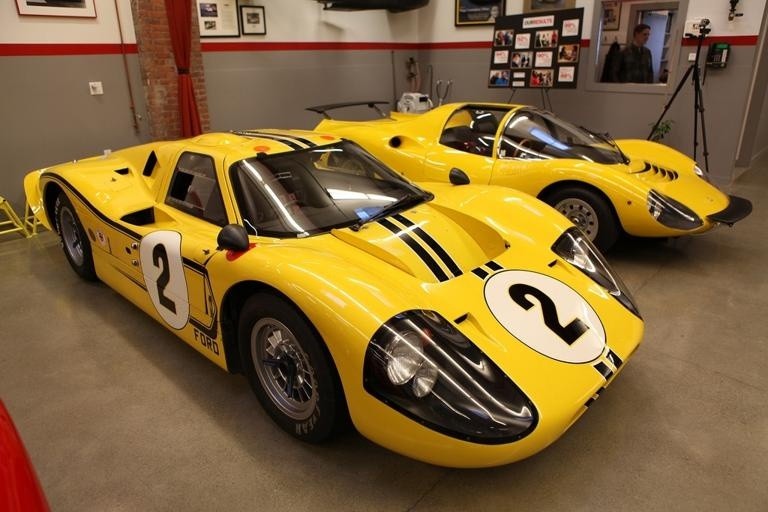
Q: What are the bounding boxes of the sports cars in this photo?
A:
[305,99,755,255]
[24,127,647,472]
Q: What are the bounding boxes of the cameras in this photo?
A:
[700,19,712,34]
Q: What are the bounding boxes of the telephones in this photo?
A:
[705,42,729,68]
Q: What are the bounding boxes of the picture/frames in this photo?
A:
[454,0,506,27]
[195,0,267,39]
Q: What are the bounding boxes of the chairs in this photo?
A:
[440,112,513,156]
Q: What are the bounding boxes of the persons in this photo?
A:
[605,23,655,84]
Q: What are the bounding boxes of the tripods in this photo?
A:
[647,35,709,173]
